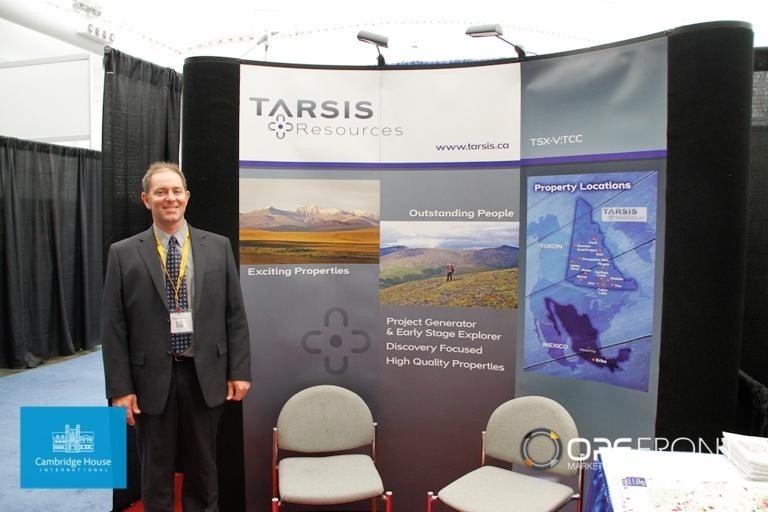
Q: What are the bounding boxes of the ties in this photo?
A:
[166,235,192,354]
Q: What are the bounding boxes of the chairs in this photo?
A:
[426,393,585,511]
[271,383,393,511]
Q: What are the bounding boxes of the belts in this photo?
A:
[173,355,193,362]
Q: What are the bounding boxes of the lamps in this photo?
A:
[465,25,528,57]
[356,31,388,65]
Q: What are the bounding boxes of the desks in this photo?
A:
[590,449,768,512]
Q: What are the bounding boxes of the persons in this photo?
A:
[101,161,251,511]
[446,264,455,282]
